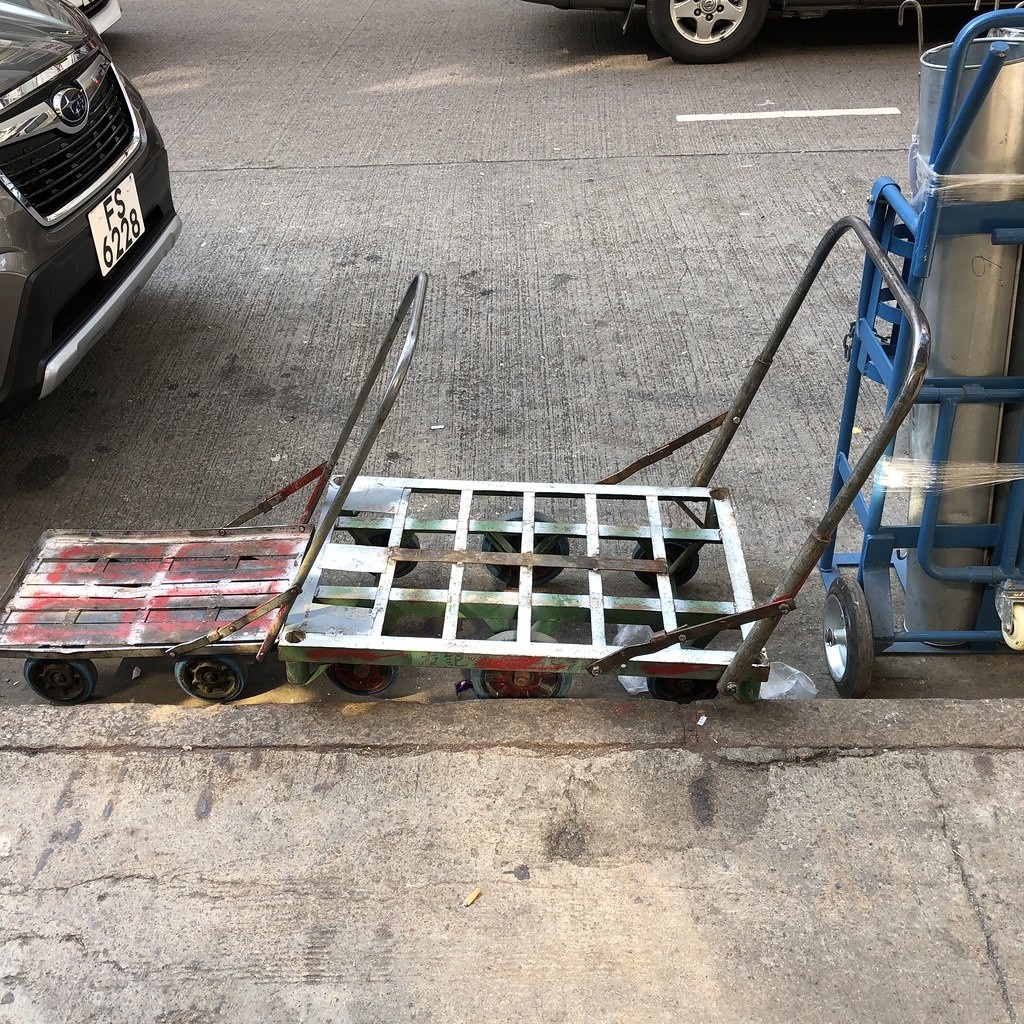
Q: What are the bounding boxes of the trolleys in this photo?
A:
[816,5,1024,697]
[273,212,933,704]
[0,271,428,704]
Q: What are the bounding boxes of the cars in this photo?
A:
[525,0,1013,66]
[0,0,185,420]
